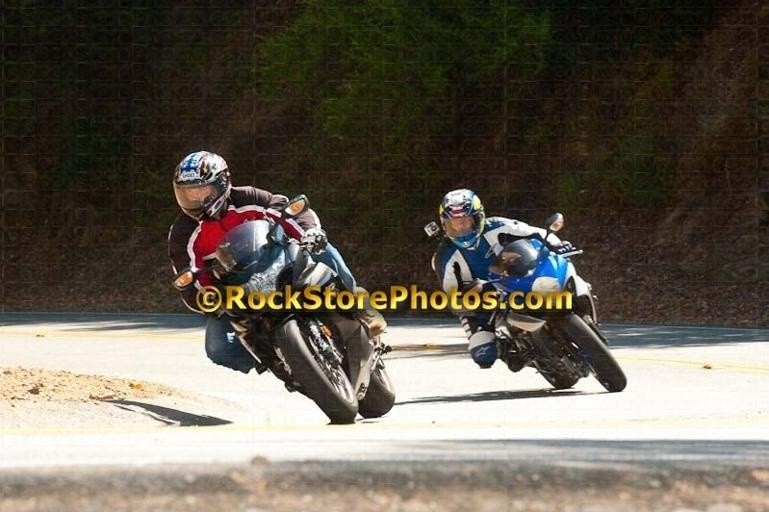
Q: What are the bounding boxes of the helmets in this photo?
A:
[438,189,485,248]
[173,151,231,221]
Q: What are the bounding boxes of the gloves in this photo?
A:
[555,242,574,257]
[300,228,328,255]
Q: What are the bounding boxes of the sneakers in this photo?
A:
[498,338,528,372]
[351,303,387,337]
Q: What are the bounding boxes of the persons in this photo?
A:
[431,188,592,373]
[168,151,387,393]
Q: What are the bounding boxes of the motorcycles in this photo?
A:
[162,192,400,425]
[458,210,631,398]
